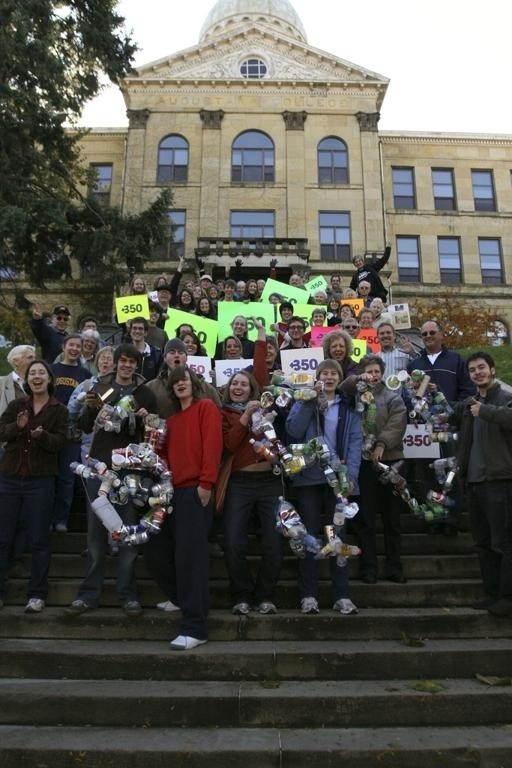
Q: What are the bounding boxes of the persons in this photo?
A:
[349,240,393,302]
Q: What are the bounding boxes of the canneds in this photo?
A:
[363,408,459,517]
[248,380,359,546]
[98,406,175,546]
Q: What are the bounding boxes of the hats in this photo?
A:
[54,306,70,315]
[200,275,212,283]
[164,338,187,355]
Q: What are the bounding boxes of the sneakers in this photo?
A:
[363,574,377,584]
[474,595,512,616]
[427,522,458,537]
[50,523,67,533]
[300,597,321,614]
[232,603,249,615]
[157,600,181,611]
[258,602,277,614]
[123,600,144,616]
[24,597,45,612]
[333,598,360,614]
[62,600,91,613]
[170,635,208,650]
[389,575,408,584]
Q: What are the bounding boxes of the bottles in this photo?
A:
[95,392,142,436]
[354,369,463,523]
[259,372,323,408]
[248,436,363,570]
[69,442,175,548]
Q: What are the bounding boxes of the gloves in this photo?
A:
[196,258,205,268]
[235,259,243,267]
[270,259,278,267]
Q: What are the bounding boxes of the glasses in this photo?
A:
[345,325,357,330]
[55,317,68,322]
[421,330,437,336]
